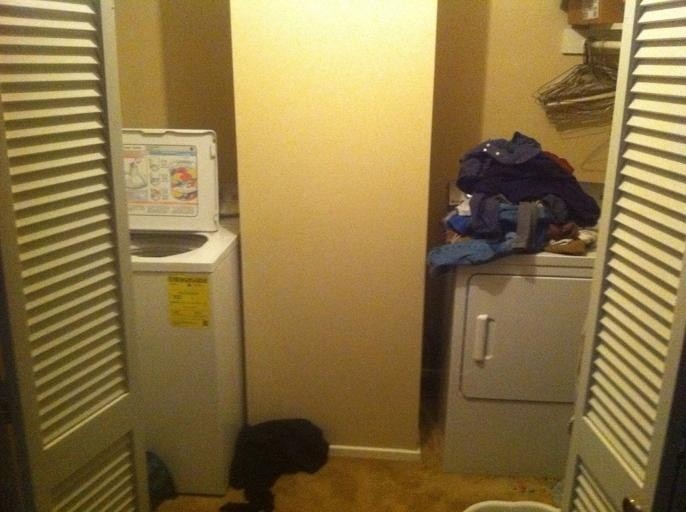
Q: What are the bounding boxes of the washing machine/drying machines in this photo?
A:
[120,125,248,498]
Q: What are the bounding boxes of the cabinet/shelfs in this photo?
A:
[439,182,603,475]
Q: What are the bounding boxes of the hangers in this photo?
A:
[531,37,617,132]
